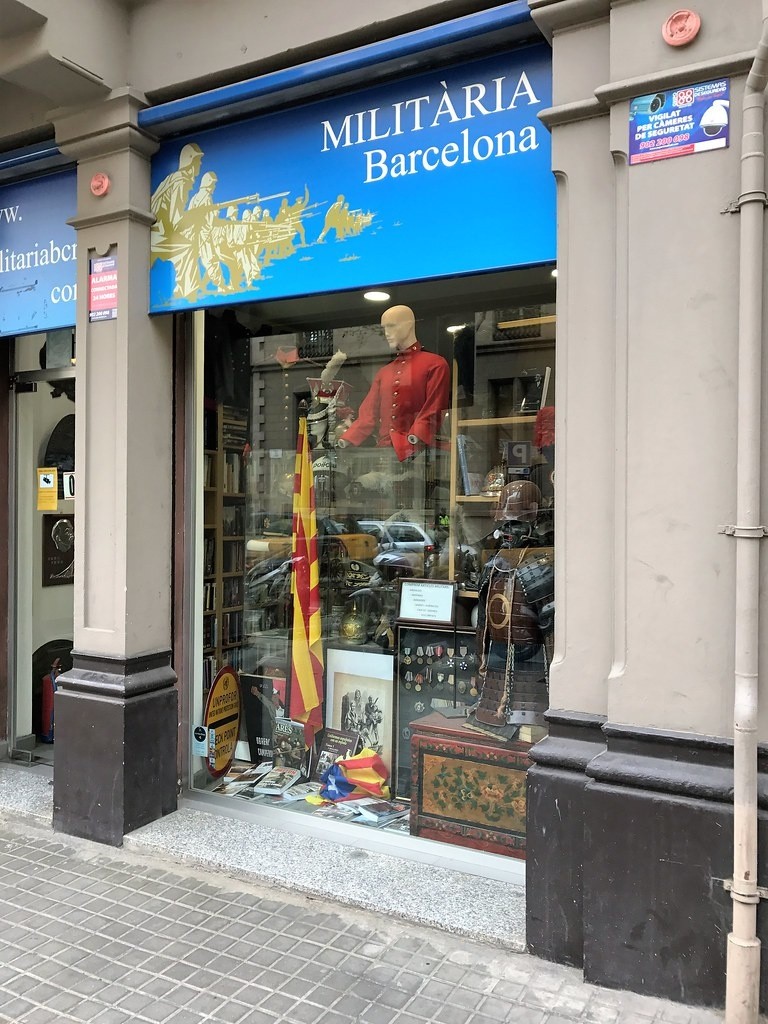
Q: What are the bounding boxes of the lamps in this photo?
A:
[362,286,394,303]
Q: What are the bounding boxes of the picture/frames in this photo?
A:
[394,577,457,627]
[320,640,398,805]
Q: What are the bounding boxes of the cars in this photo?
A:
[245,510,379,567]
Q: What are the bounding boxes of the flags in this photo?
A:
[290,417,325,751]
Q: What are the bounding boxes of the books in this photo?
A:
[223,718,410,836]
[203,406,247,689]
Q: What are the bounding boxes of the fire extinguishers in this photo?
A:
[40,657,62,743]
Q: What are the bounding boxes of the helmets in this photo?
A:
[495,480,541,522]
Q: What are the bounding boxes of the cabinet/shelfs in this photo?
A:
[449,315,556,598]
[203,402,252,718]
[410,709,537,861]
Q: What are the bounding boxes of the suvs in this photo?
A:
[359,520,449,551]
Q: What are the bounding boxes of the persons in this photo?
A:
[335,306,451,461]
[468,480,555,727]
